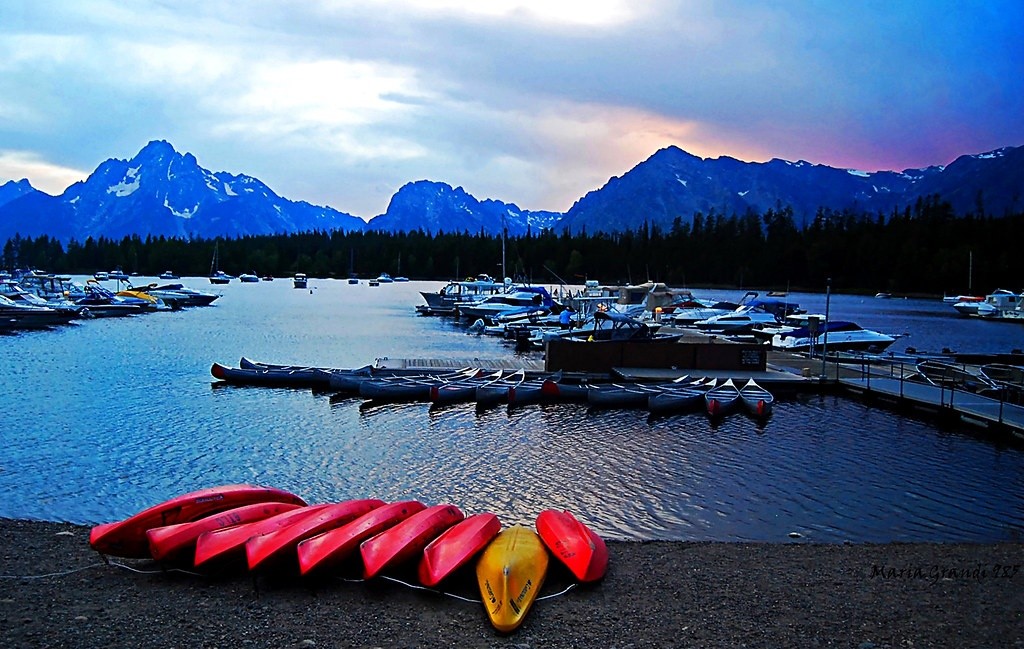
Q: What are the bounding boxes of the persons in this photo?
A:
[559,307,578,330]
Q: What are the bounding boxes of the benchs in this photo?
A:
[279,365,770,403]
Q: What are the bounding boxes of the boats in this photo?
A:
[347,271,408,287]
[419,274,684,355]
[979,363,1024,406]
[954,288,1024,321]
[211,358,774,424]
[583,281,905,349]
[0,271,274,332]
[943,295,985,305]
[88,480,609,633]
[294,272,308,288]
[915,359,999,398]
